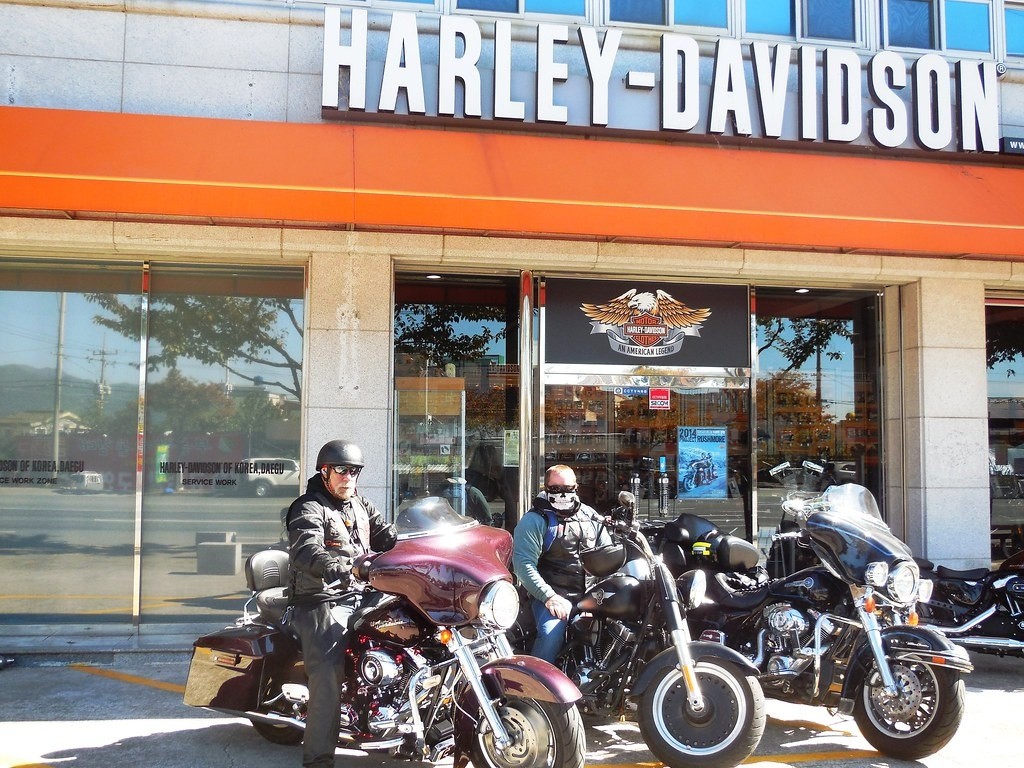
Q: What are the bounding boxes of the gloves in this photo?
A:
[322,563,348,589]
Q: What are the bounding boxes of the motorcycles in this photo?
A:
[638,482,975,763]
[907,549,1024,668]
[184,496,588,768]
[527,488,768,767]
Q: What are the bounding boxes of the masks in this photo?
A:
[548,491,577,510]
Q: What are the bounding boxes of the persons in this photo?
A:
[513,465,612,665]
[435,463,492,526]
[285,440,399,768]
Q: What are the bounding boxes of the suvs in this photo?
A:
[238,458,300,496]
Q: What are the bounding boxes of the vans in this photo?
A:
[69,470,104,493]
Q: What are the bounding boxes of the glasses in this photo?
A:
[325,464,360,478]
[546,483,577,493]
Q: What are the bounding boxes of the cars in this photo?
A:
[820,461,859,492]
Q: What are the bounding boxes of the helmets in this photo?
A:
[316,440,364,474]
[579,545,627,577]
